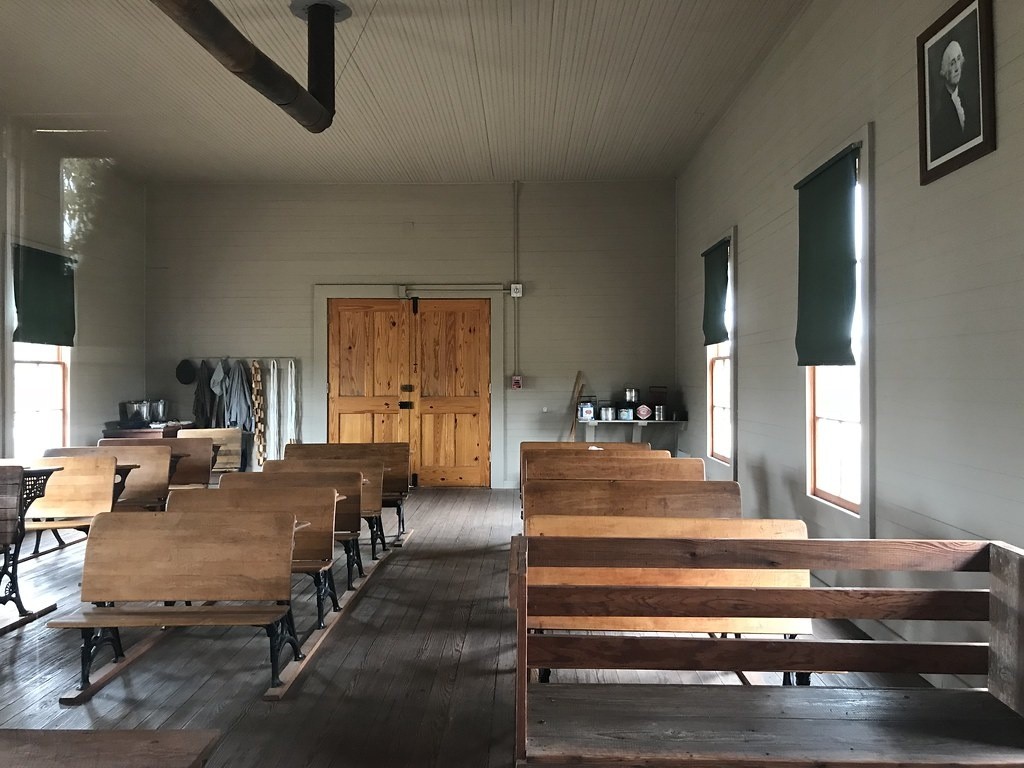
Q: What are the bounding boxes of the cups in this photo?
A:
[602,408,611,421]
[626,389,634,402]
[656,406,662,420]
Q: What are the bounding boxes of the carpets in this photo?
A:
[0,727,224,768]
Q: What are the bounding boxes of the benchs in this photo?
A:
[220,471,363,599]
[0,460,64,637]
[262,460,391,571]
[0,456,140,555]
[165,486,336,643]
[97,437,227,487]
[44,445,191,512]
[177,427,243,472]
[510,538,1024,768]
[47,511,310,705]
[525,457,706,482]
[283,442,418,546]
[522,480,743,536]
[522,449,672,481]
[520,441,652,477]
[525,514,814,686]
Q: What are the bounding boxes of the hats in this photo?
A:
[176,360,196,385]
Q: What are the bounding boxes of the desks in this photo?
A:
[101,422,194,438]
[575,418,688,443]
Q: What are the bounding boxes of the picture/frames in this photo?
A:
[916,0,997,187]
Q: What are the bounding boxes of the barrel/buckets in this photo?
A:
[128,402,149,426]
[149,400,167,423]
